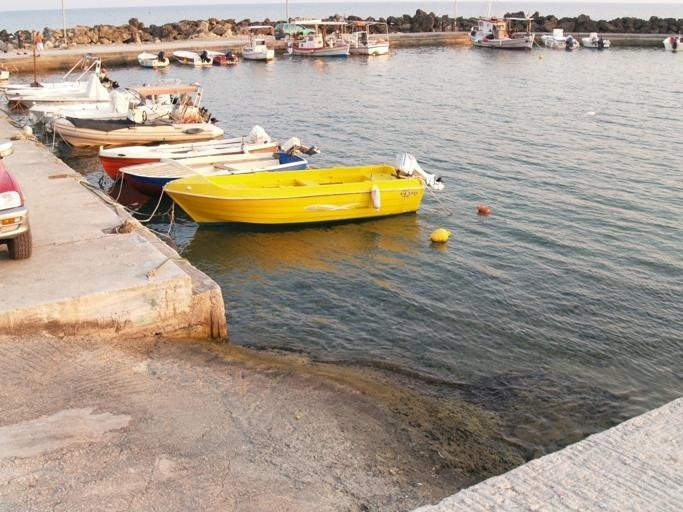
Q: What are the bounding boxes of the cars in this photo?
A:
[0,142,33,260]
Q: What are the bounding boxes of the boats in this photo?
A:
[539,28,579,49]
[661,36,682,51]
[580,32,609,48]
[468,17,535,49]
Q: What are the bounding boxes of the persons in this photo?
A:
[509,24,518,39]
[16,30,45,54]
[99,66,114,92]
